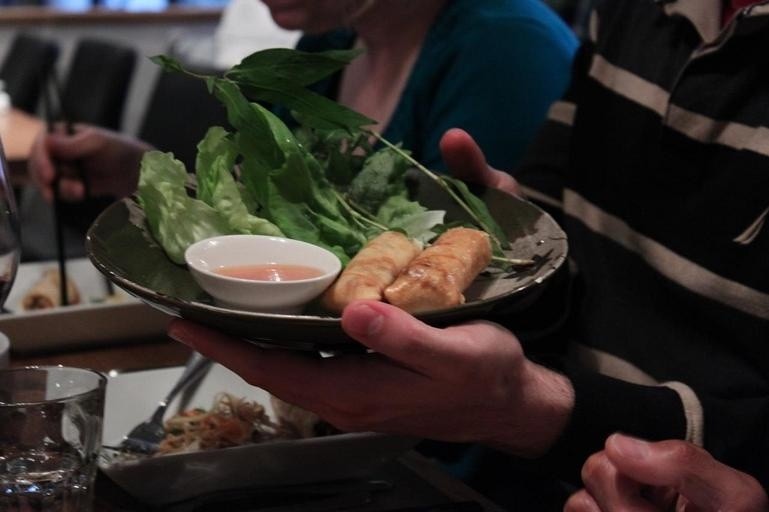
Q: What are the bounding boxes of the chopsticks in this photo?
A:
[32,57,118,308]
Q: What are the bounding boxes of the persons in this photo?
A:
[563,431,769,512]
[166,1,769,454]
[26,1,582,205]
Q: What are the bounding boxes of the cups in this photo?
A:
[0,139,25,307]
[1,363,106,512]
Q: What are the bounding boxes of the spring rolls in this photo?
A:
[320,231,423,310]
[23,269,79,313]
[383,226,490,310]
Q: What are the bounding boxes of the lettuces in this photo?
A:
[134,123,287,264]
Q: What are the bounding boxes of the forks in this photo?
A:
[113,357,215,451]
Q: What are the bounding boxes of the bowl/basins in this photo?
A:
[83,173,569,356]
[181,234,341,314]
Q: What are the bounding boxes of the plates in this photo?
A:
[1,256,178,355]
[63,361,394,503]
